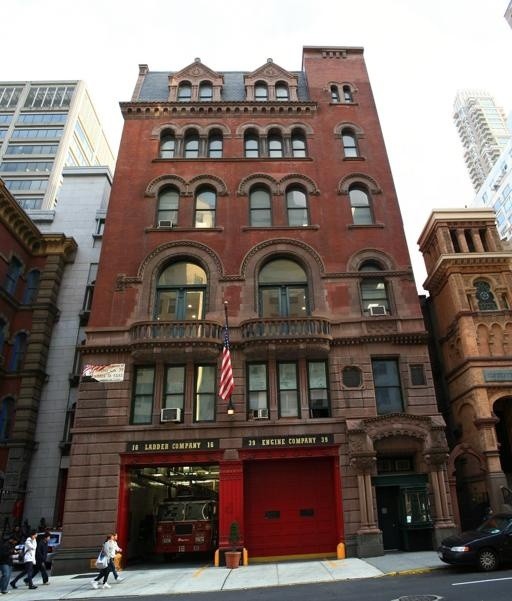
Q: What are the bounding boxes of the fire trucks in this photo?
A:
[152,484,218,563]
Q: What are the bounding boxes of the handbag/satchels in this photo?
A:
[95,556,108,569]
[18,547,24,560]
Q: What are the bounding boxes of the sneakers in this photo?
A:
[90,580,98,588]
[0,579,50,594]
[115,576,125,583]
[101,581,111,588]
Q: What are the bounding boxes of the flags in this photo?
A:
[215,326,237,404]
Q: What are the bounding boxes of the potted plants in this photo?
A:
[224,523,241,568]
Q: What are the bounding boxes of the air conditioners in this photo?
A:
[370,306,386,316]
[161,408,181,422]
[159,220,172,228]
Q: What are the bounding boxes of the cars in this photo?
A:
[436,511,511,573]
[13,527,64,570]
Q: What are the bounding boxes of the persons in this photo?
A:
[109,531,125,585]
[91,534,115,590]
[1,525,51,596]
